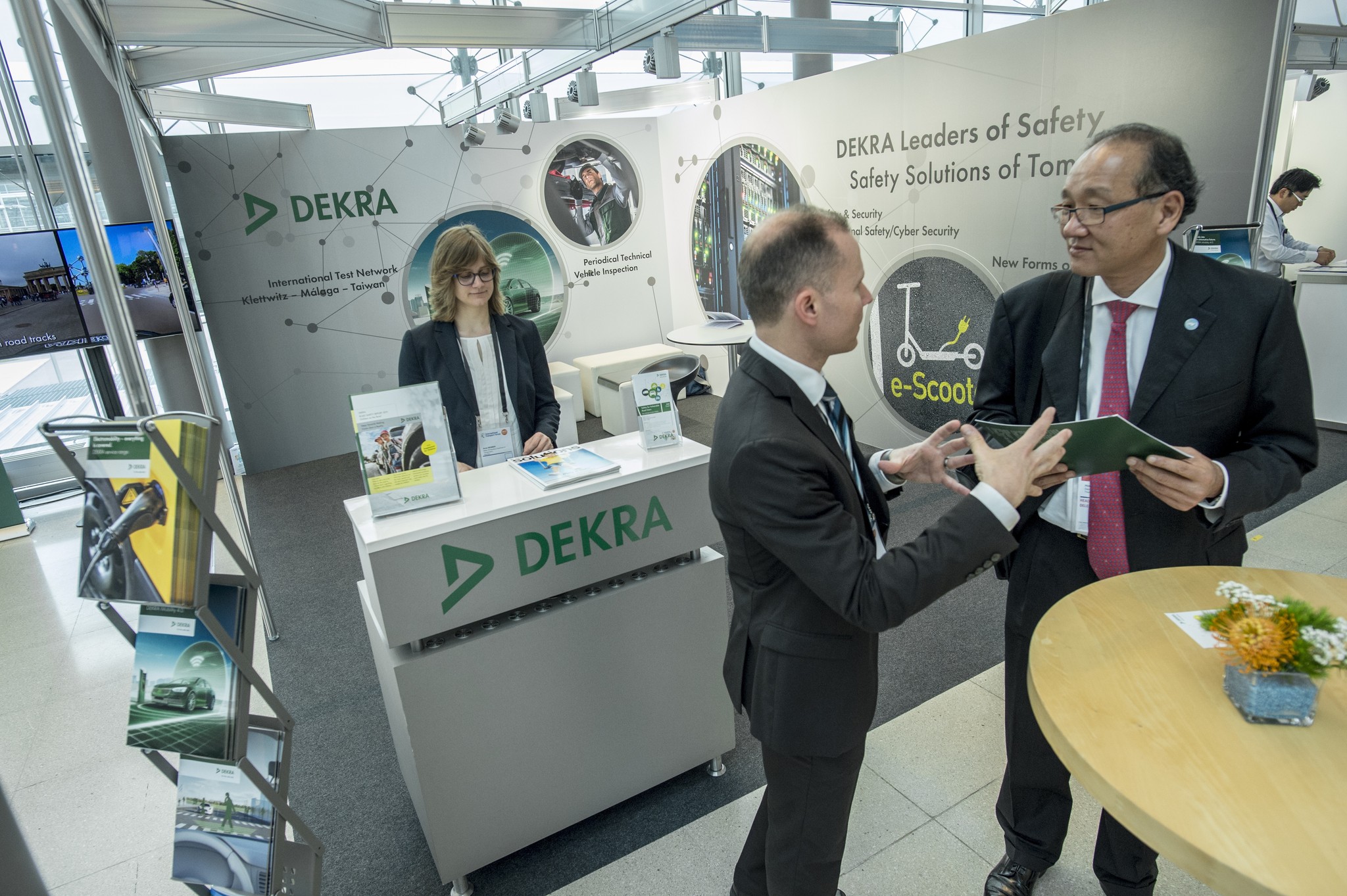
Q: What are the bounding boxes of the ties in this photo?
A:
[820,379,863,506]
[1086,299,1140,581]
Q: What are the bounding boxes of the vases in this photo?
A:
[1222,649,1327,726]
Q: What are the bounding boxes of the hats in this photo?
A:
[579,163,602,186]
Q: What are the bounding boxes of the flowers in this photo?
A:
[1194,598,1347,684]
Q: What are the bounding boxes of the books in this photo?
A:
[172,726,284,896]
[507,443,623,491]
[125,583,247,760]
[72,418,206,610]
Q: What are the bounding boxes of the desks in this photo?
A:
[343,431,735,896]
[666,320,755,378]
[1026,566,1347,896]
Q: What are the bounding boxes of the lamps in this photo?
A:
[493,103,520,135]
[523,85,550,123]
[643,25,681,79]
[567,62,599,106]
[462,118,486,147]
[1294,70,1330,101]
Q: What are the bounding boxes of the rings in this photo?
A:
[943,457,949,467]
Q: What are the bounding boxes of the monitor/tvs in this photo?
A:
[0,219,203,365]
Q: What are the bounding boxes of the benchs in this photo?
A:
[547,343,686,448]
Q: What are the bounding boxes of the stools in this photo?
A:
[638,354,701,408]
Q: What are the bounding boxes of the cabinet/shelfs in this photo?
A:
[38,410,325,896]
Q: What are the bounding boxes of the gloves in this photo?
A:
[570,178,583,200]
[584,147,601,159]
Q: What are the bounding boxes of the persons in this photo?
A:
[708,204,1071,895]
[1250,168,1336,280]
[372,430,404,474]
[562,146,631,247]
[397,225,560,474]
[957,125,1318,895]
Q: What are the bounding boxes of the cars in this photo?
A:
[197,802,214,815]
[134,282,143,288]
[168,282,198,312]
[150,676,216,713]
[401,420,433,471]
[499,278,542,316]
[375,425,404,475]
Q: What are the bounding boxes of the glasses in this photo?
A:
[1286,187,1306,203]
[1050,191,1162,226]
[453,266,494,286]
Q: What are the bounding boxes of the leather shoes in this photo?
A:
[984,852,1047,896]
[730,882,845,896]
